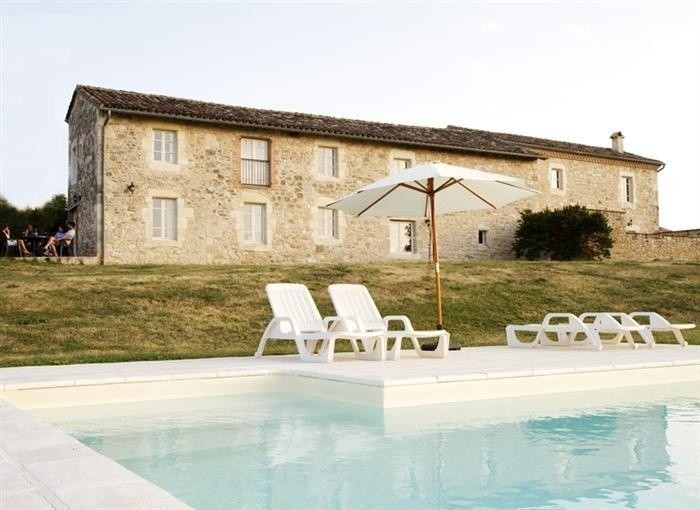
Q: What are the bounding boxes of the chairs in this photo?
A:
[314,283,451,365]
[504,306,695,353]
[1,227,78,260]
[251,282,388,370]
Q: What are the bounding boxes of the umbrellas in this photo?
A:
[324,158,544,355]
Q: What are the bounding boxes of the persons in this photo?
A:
[2,217,78,261]
